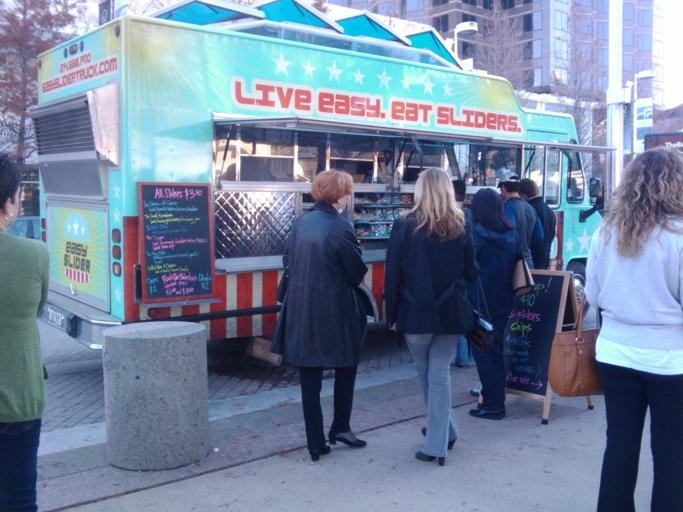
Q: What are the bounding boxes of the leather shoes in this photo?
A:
[468,407,507,420]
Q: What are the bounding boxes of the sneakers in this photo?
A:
[471,385,481,397]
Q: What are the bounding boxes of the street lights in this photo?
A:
[453,19,478,61]
[630,66,657,159]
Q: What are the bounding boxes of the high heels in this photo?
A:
[415,450,445,467]
[328,426,367,448]
[421,426,456,449]
[309,445,331,461]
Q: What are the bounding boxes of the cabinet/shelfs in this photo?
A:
[235,125,447,189]
[353,201,419,243]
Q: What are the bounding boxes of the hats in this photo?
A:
[497,171,521,188]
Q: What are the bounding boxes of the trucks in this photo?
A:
[33,11,605,383]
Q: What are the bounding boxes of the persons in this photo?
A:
[0,150,50,511]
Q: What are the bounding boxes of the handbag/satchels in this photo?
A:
[511,258,537,297]
[275,272,292,304]
[544,327,610,399]
[469,306,498,353]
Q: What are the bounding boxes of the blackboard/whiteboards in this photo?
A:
[502,270,568,402]
[136,182,218,303]
